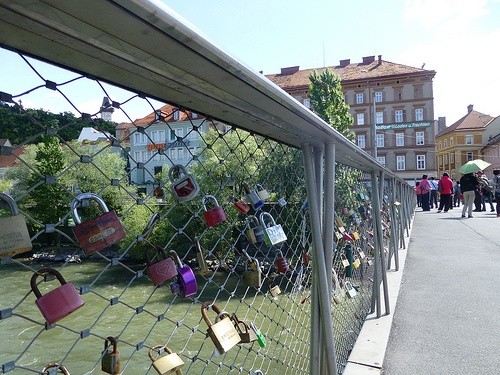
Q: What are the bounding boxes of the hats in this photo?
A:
[476,171,483,173]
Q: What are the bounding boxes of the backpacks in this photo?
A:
[456,185,460,195]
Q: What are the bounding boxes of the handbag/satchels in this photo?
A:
[483,186,491,197]
[438,180,442,192]
[436,186,439,190]
[426,182,431,190]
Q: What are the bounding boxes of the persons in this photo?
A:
[415,169,500,218]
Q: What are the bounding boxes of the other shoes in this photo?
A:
[468,216,473,218]
[491,209,495,212]
[462,215,465,217]
[436,210,441,213]
[481,210,486,211]
[472,209,478,211]
[497,214,500,217]
[444,211,446,213]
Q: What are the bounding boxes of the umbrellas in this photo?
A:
[457,159,492,174]
